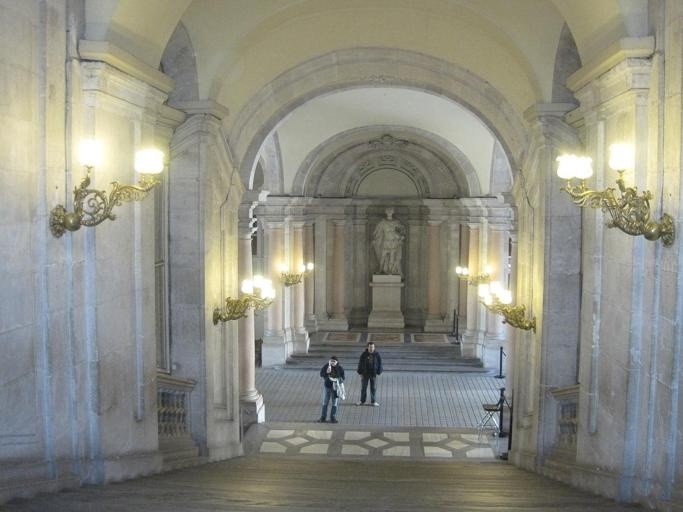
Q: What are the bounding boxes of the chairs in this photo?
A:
[318,416,324,422]
[372,402,379,406]
[356,400,362,405]
[331,417,337,422]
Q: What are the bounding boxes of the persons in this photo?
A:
[316,356,346,423]
[369,207,407,275]
[355,341,383,407]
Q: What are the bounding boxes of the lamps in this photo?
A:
[477,401,499,433]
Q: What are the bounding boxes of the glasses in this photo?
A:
[279,263,313,286]
[49,142,164,238]
[556,146,675,248]
[457,266,491,286]
[213,277,276,325]
[478,283,536,334]
